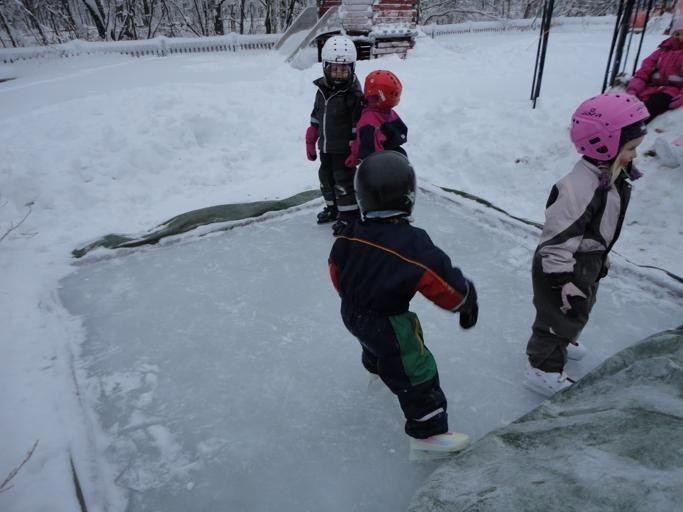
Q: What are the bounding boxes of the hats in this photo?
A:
[670,15,683,35]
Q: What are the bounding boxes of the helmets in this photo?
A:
[570,93,651,162]
[353,150,414,222]
[321,36,357,89]
[364,71,402,112]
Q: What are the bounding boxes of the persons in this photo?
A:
[626,17,683,134]
[327,150,478,452]
[344,69,408,169]
[524,93,650,399]
[305,36,365,232]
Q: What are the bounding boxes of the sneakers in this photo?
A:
[524,363,577,392]
[566,339,586,361]
[410,429,471,450]
[332,221,350,230]
[317,208,337,221]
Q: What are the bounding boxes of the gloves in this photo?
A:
[344,140,359,168]
[457,282,478,331]
[306,127,318,161]
[669,94,683,109]
[546,274,588,320]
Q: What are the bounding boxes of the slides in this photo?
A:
[263,4,338,65]
